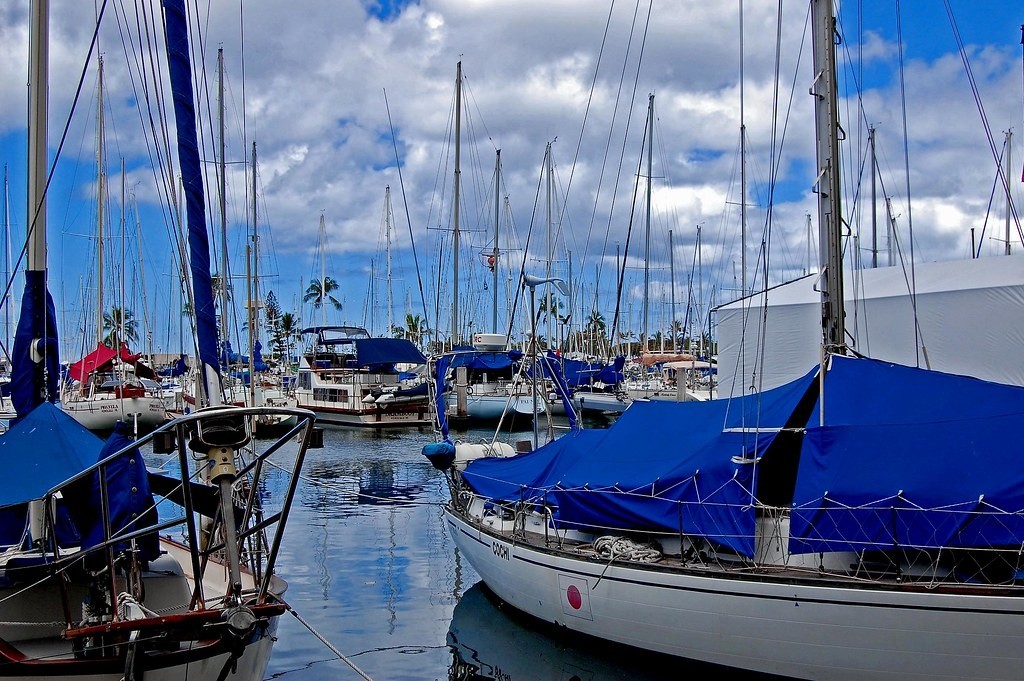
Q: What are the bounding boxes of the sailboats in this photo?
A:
[0,0,316,681]
[423,0,1023,681]
[0,0,754,447]
